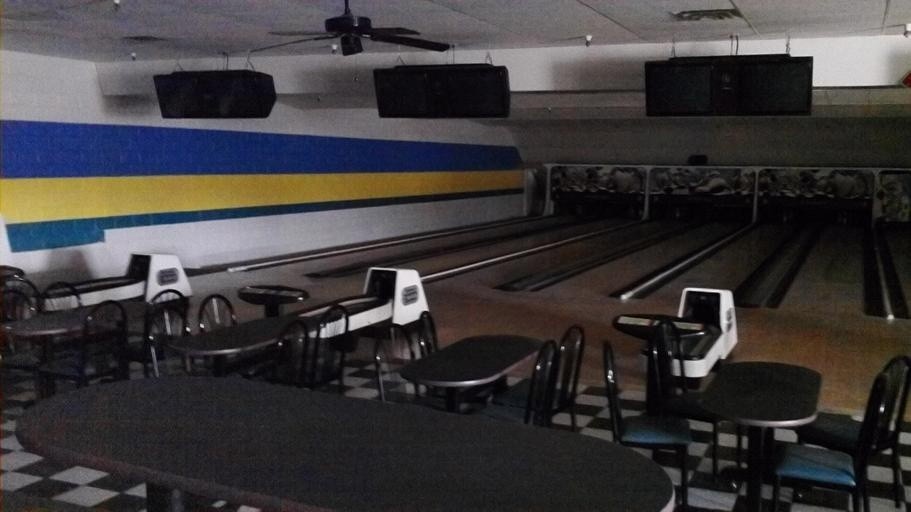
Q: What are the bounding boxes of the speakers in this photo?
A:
[156,71,278,119]
[643,56,813,115]
[372,65,512,119]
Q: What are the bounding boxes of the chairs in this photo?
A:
[643,316,732,479]
[795,356,910,510]
[600,340,694,500]
[738,370,887,511]
[1,267,585,430]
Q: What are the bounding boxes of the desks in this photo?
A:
[687,362,825,493]
[14,374,678,512]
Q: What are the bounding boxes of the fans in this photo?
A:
[239,1,462,57]
